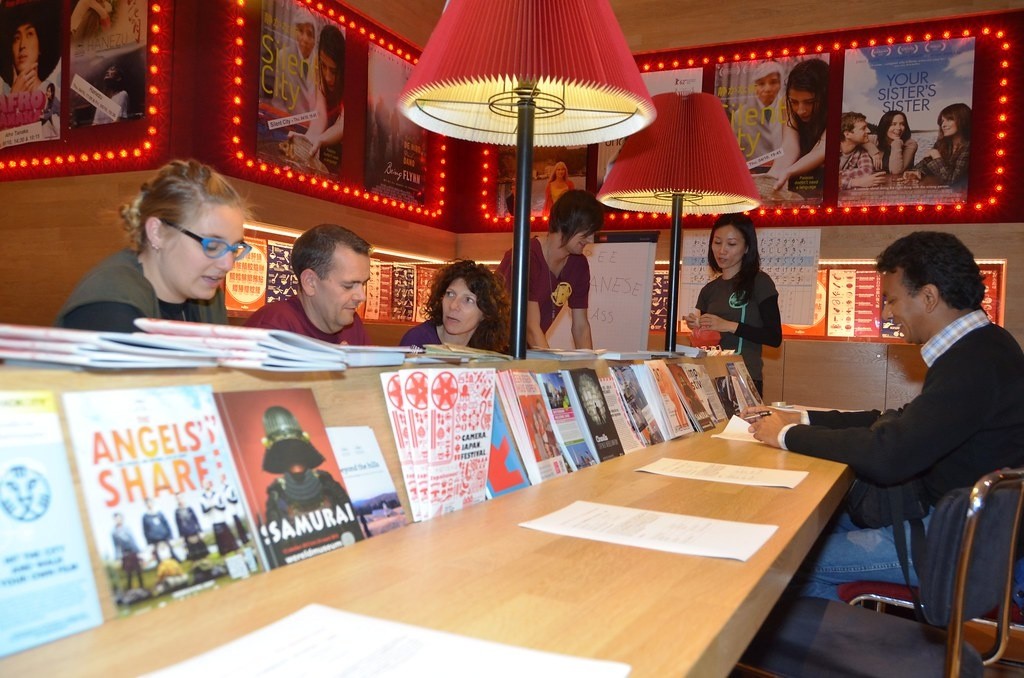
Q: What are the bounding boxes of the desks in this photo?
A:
[0,404,860,678]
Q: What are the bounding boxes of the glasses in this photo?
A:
[160,216,252,261]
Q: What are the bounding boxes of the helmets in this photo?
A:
[261,406,326,475]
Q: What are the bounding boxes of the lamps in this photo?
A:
[595,92,763,351]
[399,0,655,361]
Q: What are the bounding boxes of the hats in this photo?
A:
[0,0,60,89]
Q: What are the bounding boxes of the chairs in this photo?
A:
[740,468,1024,677]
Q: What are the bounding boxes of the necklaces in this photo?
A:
[547,240,564,318]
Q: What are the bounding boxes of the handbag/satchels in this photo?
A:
[688,326,721,347]
[846,408,926,625]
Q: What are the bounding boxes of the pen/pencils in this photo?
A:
[744,410,772,421]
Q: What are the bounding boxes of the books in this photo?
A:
[0,369,525,657]
[712,361,765,418]
[600,359,728,453]
[496,368,624,485]
[0,315,514,372]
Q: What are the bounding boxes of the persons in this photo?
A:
[0,4,62,123]
[682,213,782,400]
[272,8,346,159]
[492,189,603,349]
[399,260,510,352]
[839,103,972,189]
[731,58,829,197]
[52,158,251,332]
[740,231,1024,614]
[244,224,372,345]
[542,162,575,217]
[92,65,128,125]
[71,0,112,40]
